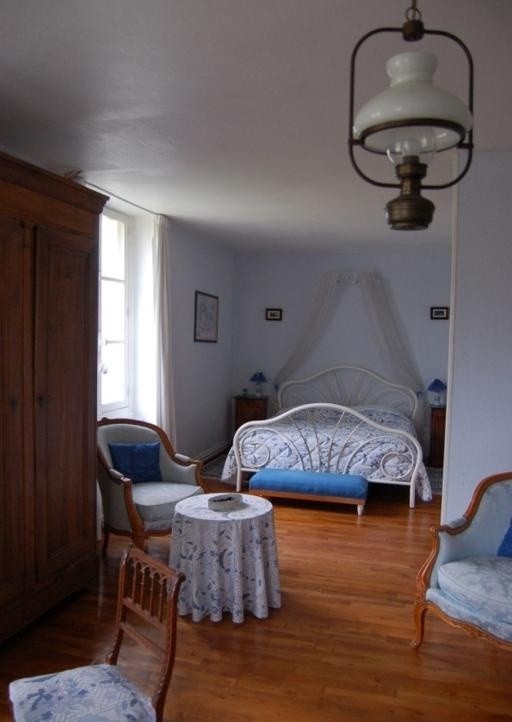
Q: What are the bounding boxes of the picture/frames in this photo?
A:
[266,308,283,321]
[193,290,219,343]
[430,307,448,320]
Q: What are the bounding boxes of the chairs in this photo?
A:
[408,471,512,651]
[9,545,186,722]
[96,416,207,571]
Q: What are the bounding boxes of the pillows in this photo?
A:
[108,441,164,483]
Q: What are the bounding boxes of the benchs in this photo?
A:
[249,469,370,516]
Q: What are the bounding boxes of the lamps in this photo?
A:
[346,1,474,232]
[249,372,267,396]
[427,378,446,406]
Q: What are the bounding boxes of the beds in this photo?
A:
[231,365,424,509]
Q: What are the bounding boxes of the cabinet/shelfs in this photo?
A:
[0,148,110,648]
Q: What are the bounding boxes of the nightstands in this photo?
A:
[233,395,268,431]
[429,404,445,468]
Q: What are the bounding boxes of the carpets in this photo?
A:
[200,453,228,480]
[422,465,442,495]
